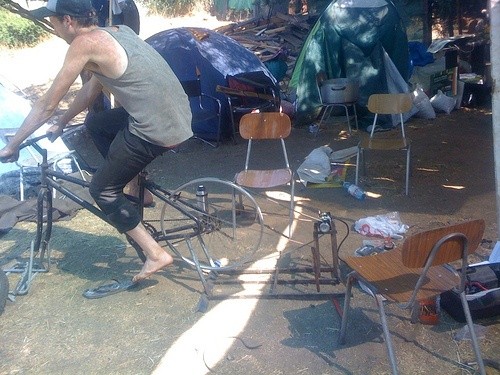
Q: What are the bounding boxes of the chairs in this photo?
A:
[358,93,415,196]
[314,71,358,139]
[0,128,87,201]
[233,111,294,240]
[339,219,488,375]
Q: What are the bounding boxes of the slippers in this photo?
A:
[123,194,155,207]
[82,278,139,298]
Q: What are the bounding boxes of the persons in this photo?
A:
[0,0,195,283]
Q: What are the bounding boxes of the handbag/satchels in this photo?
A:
[440,262,500,323]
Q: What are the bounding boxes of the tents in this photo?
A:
[283,0,418,134]
[140,26,282,142]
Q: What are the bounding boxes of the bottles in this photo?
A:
[339,181,367,201]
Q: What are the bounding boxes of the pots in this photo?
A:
[318,79,359,104]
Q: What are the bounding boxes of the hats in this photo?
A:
[31,0,93,17]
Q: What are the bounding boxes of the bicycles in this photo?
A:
[15,132,265,273]
